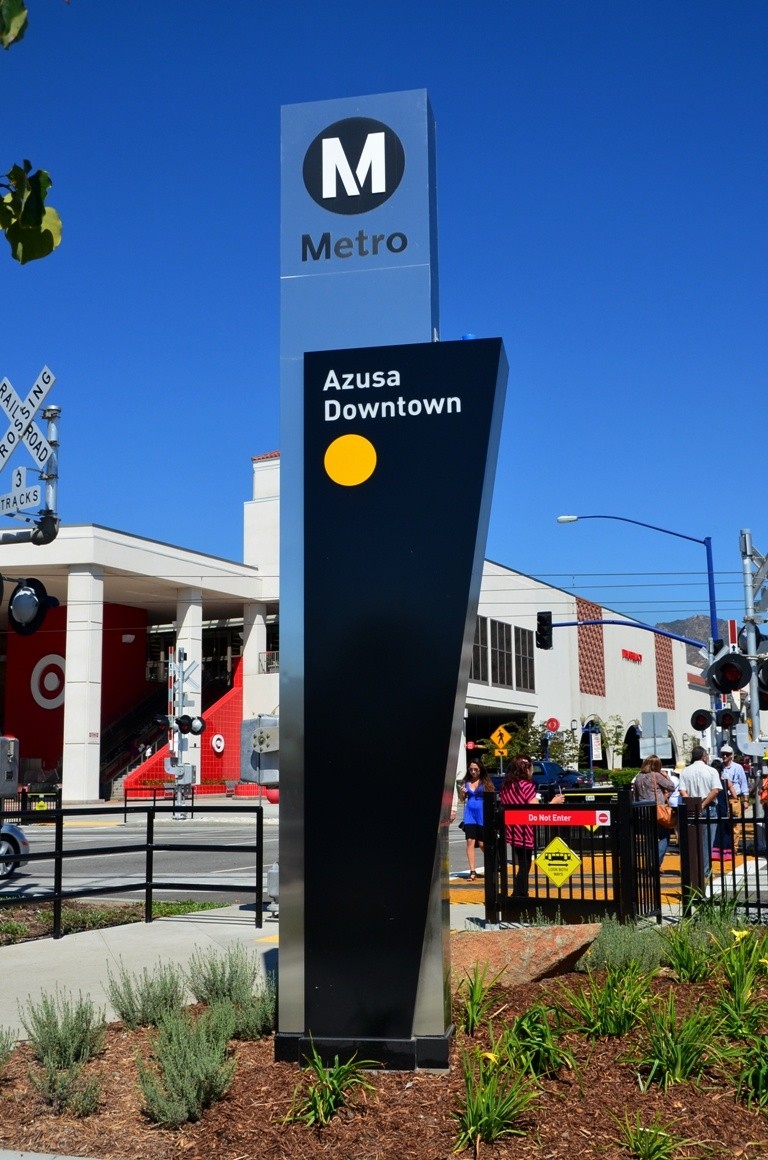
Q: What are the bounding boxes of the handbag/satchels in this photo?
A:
[727,808,730,817]
[458,821,466,832]
[657,804,678,828]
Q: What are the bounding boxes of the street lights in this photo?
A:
[555,513,723,756]
[579,712,600,782]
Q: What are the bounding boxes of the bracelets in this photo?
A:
[744,800,749,803]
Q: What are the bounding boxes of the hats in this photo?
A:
[762,749,768,760]
[720,744,733,753]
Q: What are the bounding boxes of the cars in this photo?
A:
[487,759,593,791]
[0,824,31,880]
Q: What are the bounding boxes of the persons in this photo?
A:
[634,753,675,877]
[708,745,751,862]
[662,768,683,847]
[459,762,496,881]
[679,745,724,887]
[127,735,152,764]
[500,753,565,897]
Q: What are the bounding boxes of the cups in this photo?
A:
[555,794,564,803]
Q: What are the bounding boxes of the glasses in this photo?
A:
[721,753,730,756]
[468,767,478,771]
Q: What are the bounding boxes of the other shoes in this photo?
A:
[467,871,478,882]
[712,848,721,861]
[723,849,734,861]
[659,867,665,874]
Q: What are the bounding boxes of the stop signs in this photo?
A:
[598,814,608,824]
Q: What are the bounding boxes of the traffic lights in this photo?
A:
[691,709,712,732]
[758,688,768,710]
[714,653,752,690]
[535,610,552,650]
[174,715,192,734]
[718,708,738,727]
[10,576,60,636]
